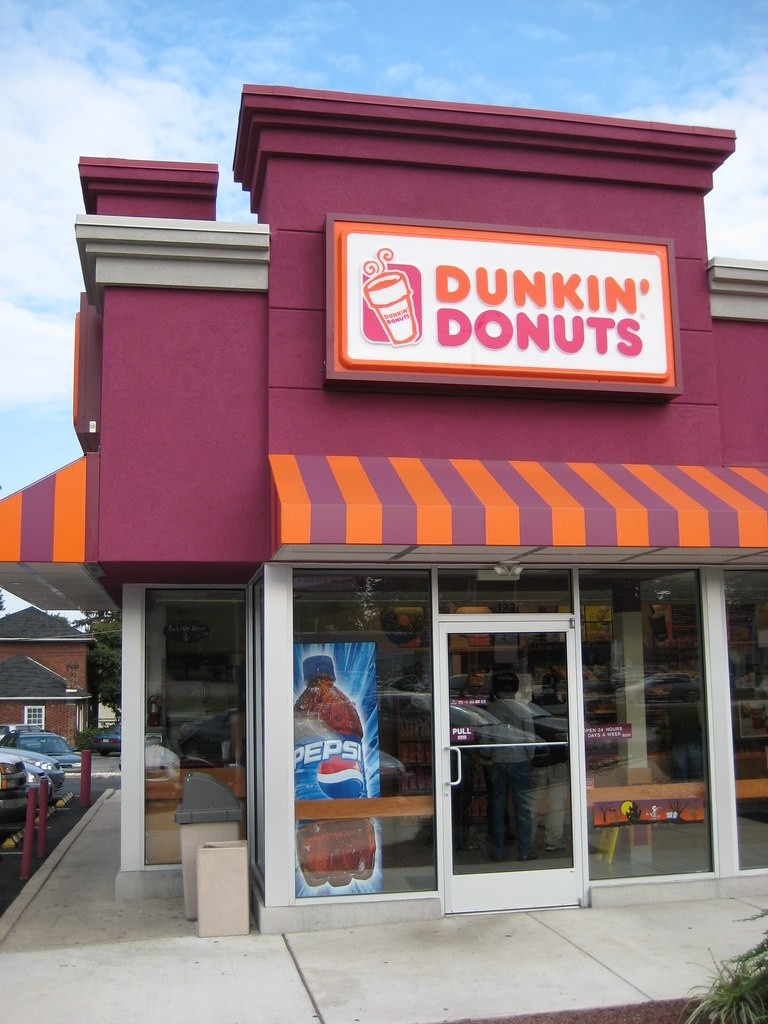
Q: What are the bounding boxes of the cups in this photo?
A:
[364,270,419,345]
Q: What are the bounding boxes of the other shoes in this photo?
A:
[493,852,508,862]
[517,852,538,861]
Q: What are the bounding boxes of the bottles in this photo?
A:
[294,656,377,888]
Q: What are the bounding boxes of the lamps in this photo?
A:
[490,560,523,576]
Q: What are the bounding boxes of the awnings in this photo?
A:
[267,455,767,558]
[1,453,118,610]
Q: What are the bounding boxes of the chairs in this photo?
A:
[41,742,56,751]
[19,744,26,748]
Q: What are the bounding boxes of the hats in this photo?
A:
[495,672,519,692]
[8,725,17,732]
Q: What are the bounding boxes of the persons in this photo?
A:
[480,672,536,863]
[529,648,704,852]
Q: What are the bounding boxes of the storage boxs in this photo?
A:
[196,840,250,937]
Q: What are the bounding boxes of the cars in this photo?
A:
[0,747,67,791]
[476,697,594,763]
[26,763,55,810]
[0,751,28,811]
[616,670,705,703]
[374,688,553,766]
[180,707,405,801]
[0,722,82,774]
[92,722,121,755]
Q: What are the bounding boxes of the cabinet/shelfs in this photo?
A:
[379,691,493,836]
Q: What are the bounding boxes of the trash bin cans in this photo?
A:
[173,772,248,918]
[194,840,250,937]
